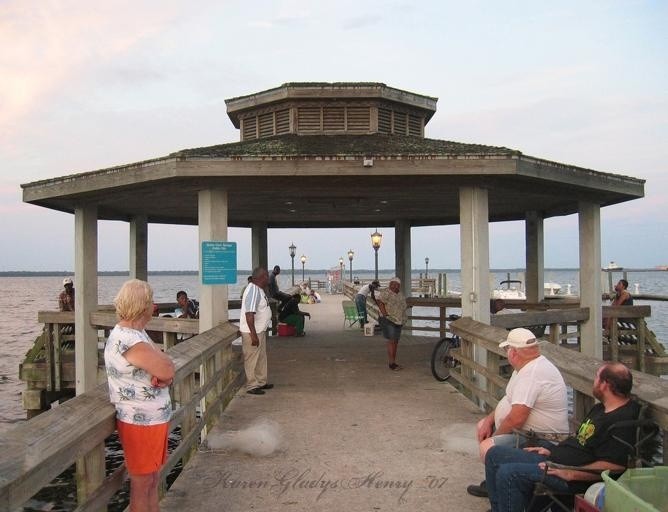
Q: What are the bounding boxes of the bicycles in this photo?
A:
[429,312,465,383]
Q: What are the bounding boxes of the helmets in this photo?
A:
[498,328,538,350]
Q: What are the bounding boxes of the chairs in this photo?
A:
[342,301,365,330]
[512,394,659,512]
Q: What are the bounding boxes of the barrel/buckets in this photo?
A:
[364,323,375,336]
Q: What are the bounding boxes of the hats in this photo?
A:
[62,277,73,288]
[372,279,381,287]
[391,277,402,284]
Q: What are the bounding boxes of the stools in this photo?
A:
[277,322,295,336]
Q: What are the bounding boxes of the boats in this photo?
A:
[492,274,561,341]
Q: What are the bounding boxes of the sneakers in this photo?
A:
[261,383,274,390]
[467,480,488,498]
[389,362,403,371]
[246,387,266,395]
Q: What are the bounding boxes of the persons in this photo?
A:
[104,279,176,512]
[175,291,198,342]
[376,277,408,371]
[467,327,569,497]
[237,266,322,394]
[485,362,640,512]
[57,277,74,310]
[354,280,381,329]
[603,279,633,336]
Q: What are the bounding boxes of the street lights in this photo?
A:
[369,228,382,281]
[347,247,353,283]
[424,256,430,276]
[300,254,307,281]
[338,254,344,281]
[289,241,297,286]
